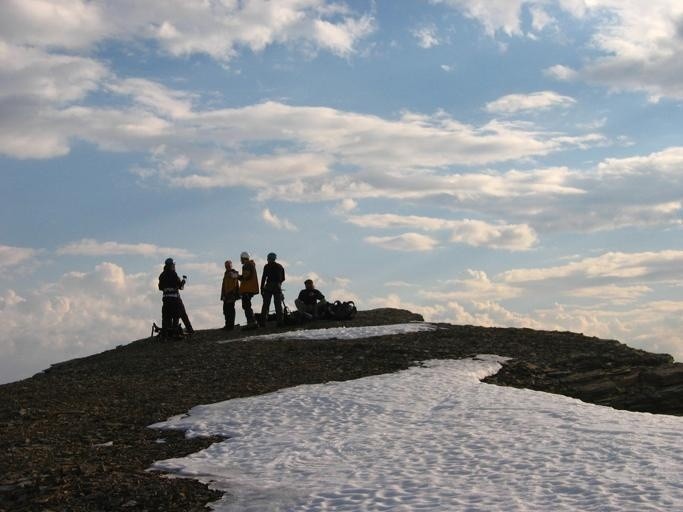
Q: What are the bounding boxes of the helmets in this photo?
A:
[267,253,277,261]
[165,258,175,264]
[240,252,250,258]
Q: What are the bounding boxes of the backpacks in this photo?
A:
[326,300,356,320]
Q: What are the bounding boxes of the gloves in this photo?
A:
[231,272,238,278]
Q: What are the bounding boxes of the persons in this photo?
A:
[220,260,238,331]
[158,258,195,334]
[231,252,259,331]
[295,279,325,317]
[261,252,285,328]
